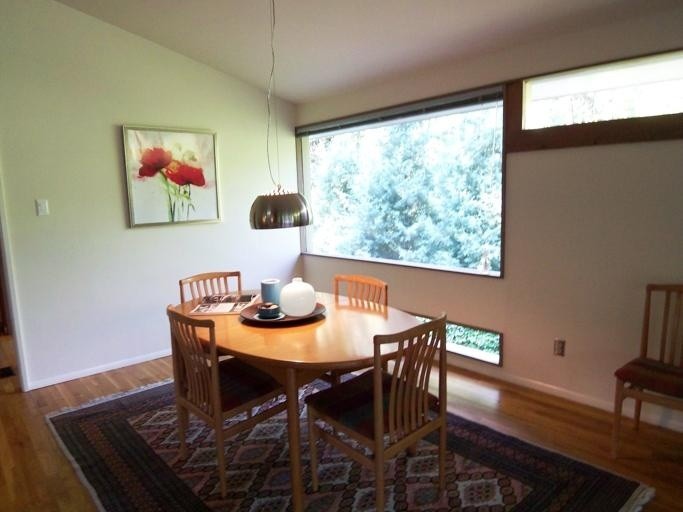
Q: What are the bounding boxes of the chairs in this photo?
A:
[177,269,255,433]
[167,303,299,498]
[302,311,449,510]
[611,281,683,440]
[316,273,391,450]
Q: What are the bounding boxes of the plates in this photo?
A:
[252,314,286,321]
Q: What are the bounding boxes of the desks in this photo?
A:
[172,286,431,510]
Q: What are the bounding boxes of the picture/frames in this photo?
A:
[122,124,223,229]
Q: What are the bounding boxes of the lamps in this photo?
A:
[248,0,312,231]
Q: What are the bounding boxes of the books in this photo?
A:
[189,294,259,315]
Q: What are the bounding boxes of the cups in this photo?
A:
[261,279,279,303]
[257,303,280,319]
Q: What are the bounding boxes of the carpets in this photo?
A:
[42,372,658,511]
